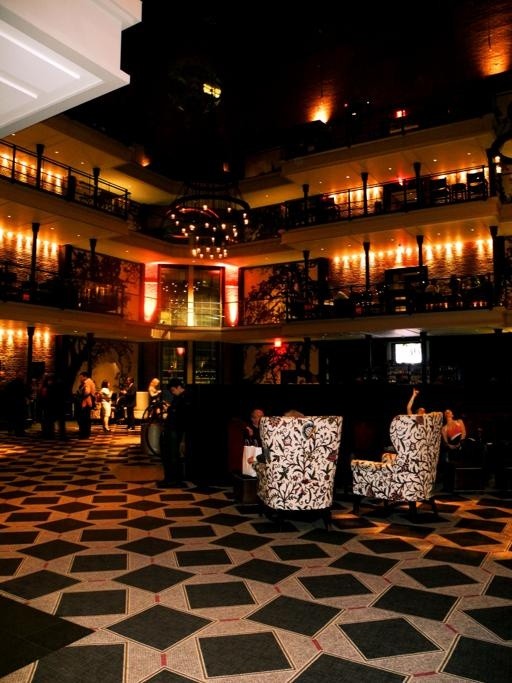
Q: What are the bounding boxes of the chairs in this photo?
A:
[350,411,443,523]
[429,171,488,204]
[254,416,344,531]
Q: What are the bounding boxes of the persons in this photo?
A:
[0,358,164,440]
[247,453,266,465]
[441,408,466,462]
[242,407,265,444]
[465,423,511,489]
[326,273,488,309]
[406,387,426,414]
[156,377,196,488]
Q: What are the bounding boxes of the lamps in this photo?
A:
[168,164,253,260]
